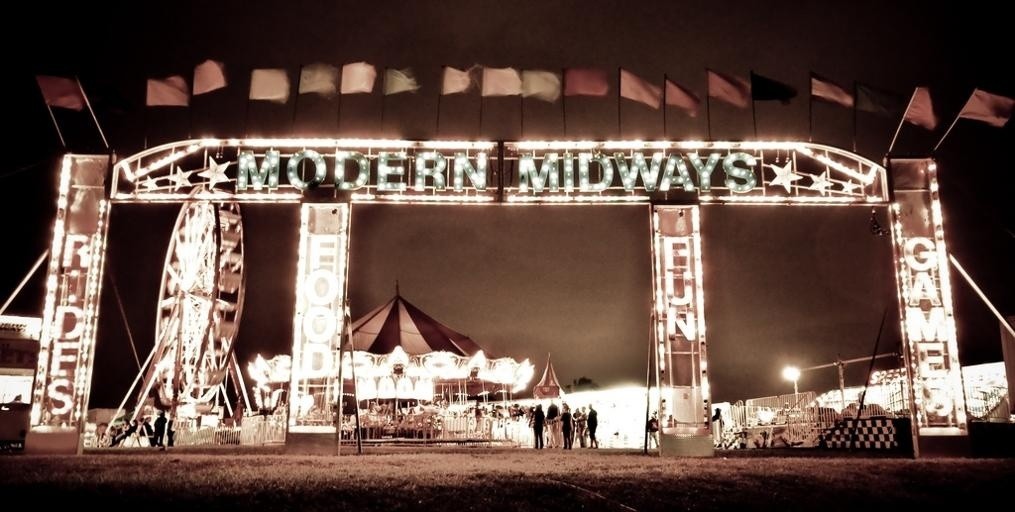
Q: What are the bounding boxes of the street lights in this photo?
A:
[782,365,801,413]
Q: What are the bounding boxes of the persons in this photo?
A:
[93,412,176,448]
[645,416,662,450]
[666,415,680,429]
[712,406,726,433]
[474,399,604,452]
